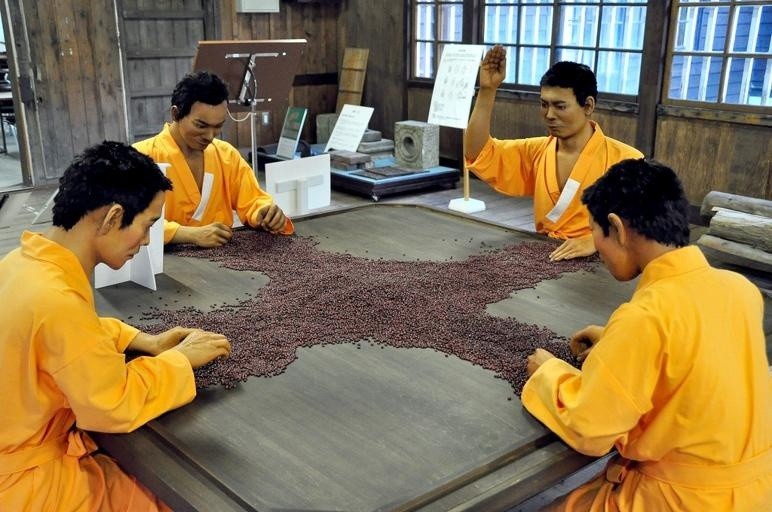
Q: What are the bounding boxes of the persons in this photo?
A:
[517,157,772,512]
[0,139,234,512]
[463,42,647,265]
[128,69,295,250]
